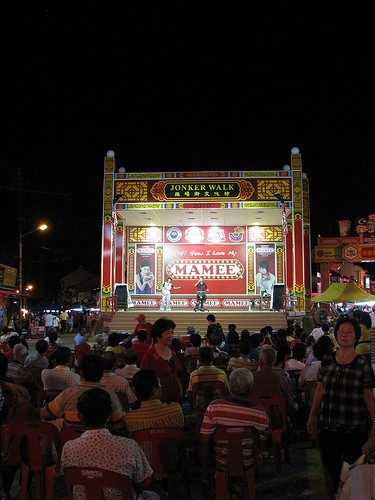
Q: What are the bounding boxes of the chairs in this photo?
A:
[24,367,46,390]
[11,421,60,500]
[63,467,134,500]
[132,427,191,500]
[241,392,289,477]
[192,381,228,420]
[304,380,317,448]
[210,426,258,500]
[36,390,62,407]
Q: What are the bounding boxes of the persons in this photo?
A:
[310,322,324,342]
[250,347,298,424]
[353,310,372,355]
[58,387,161,500]
[286,343,306,373]
[322,324,339,352]
[181,315,307,398]
[0,307,153,500]
[171,338,182,360]
[256,261,275,292]
[370,305,375,328]
[142,318,182,407]
[124,370,188,484]
[307,318,375,500]
[200,368,272,486]
[194,278,209,311]
[160,278,173,310]
[298,338,334,389]
[136,260,154,294]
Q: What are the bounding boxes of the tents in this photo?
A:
[310,282,375,308]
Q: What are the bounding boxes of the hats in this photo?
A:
[136,314,145,320]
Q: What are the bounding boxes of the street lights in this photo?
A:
[19,223,49,338]
[24,285,33,326]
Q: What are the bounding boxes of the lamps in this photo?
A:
[114,194,122,201]
[274,193,284,206]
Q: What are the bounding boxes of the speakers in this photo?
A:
[115,285,127,309]
[273,285,284,308]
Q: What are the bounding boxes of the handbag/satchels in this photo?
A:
[335,454,375,500]
